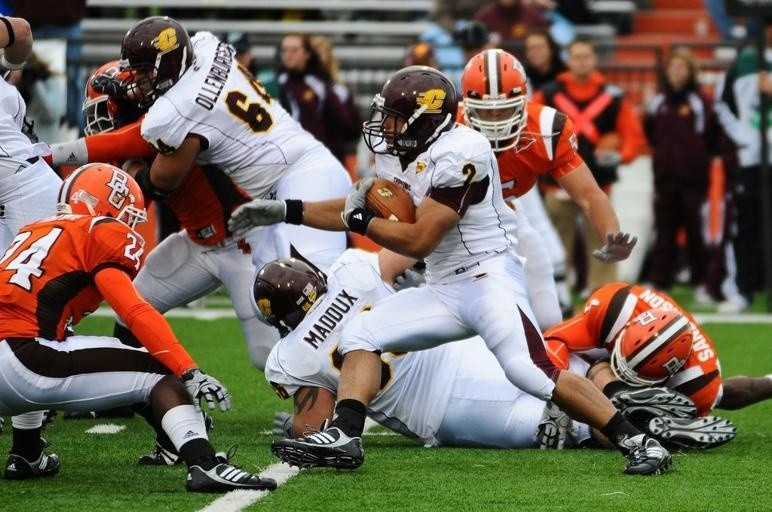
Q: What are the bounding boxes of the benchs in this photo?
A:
[596,3,738,110]
[83,2,438,116]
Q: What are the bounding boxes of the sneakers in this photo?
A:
[3,437,61,479]
[138,408,215,466]
[186,444,277,493]
[271,419,365,471]
[609,386,737,475]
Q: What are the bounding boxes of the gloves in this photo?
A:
[340,176,373,232]
[180,367,232,414]
[533,399,577,451]
[226,199,304,242]
[271,411,295,438]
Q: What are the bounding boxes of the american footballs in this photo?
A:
[357,178,415,224]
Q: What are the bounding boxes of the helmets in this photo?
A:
[81,60,132,138]
[117,16,193,112]
[362,64,457,159]
[251,257,327,337]
[460,46,527,154]
[610,307,693,389]
[56,161,149,232]
